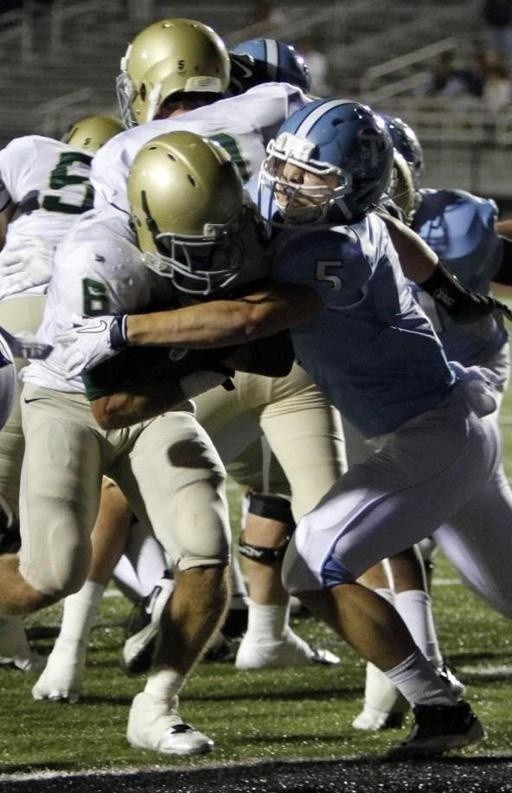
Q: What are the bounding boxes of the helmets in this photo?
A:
[125,130,274,298]
[234,36,313,94]
[61,113,126,154]
[260,95,424,227]
[112,17,234,131]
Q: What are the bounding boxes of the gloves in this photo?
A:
[52,311,134,382]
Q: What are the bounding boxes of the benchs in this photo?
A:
[4,0,512,151]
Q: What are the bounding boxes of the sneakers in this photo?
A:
[0,650,49,674]
[350,641,489,764]
[228,591,302,620]
[202,631,238,664]
[122,566,177,675]
[125,690,218,758]
[234,625,342,673]
[31,638,89,705]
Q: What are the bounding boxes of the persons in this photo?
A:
[0,15,511,762]
